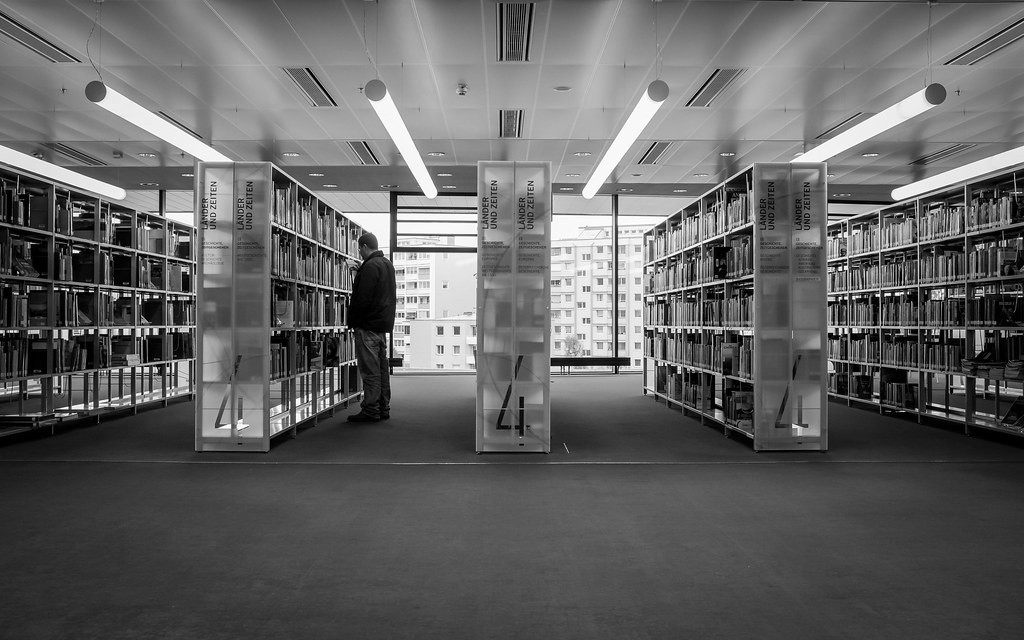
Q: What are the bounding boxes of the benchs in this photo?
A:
[552,356,631,375]
[388,358,402,375]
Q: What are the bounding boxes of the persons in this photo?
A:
[347,233,396,424]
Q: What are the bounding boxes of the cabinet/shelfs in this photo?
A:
[829,161,1023,437]
[639,159,829,453]
[0,161,197,439]
[192,160,372,455]
[477,160,552,454]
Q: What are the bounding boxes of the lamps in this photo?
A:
[363,79,439,201]
[581,80,669,199]
[789,82,947,163]
[0,143,128,201]
[890,145,1024,202]
[85,80,235,162]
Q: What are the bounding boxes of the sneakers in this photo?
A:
[347,410,390,422]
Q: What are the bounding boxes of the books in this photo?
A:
[644,170,823,437]
[825,184,1024,432]
[203,178,369,395]
[1,177,197,382]
